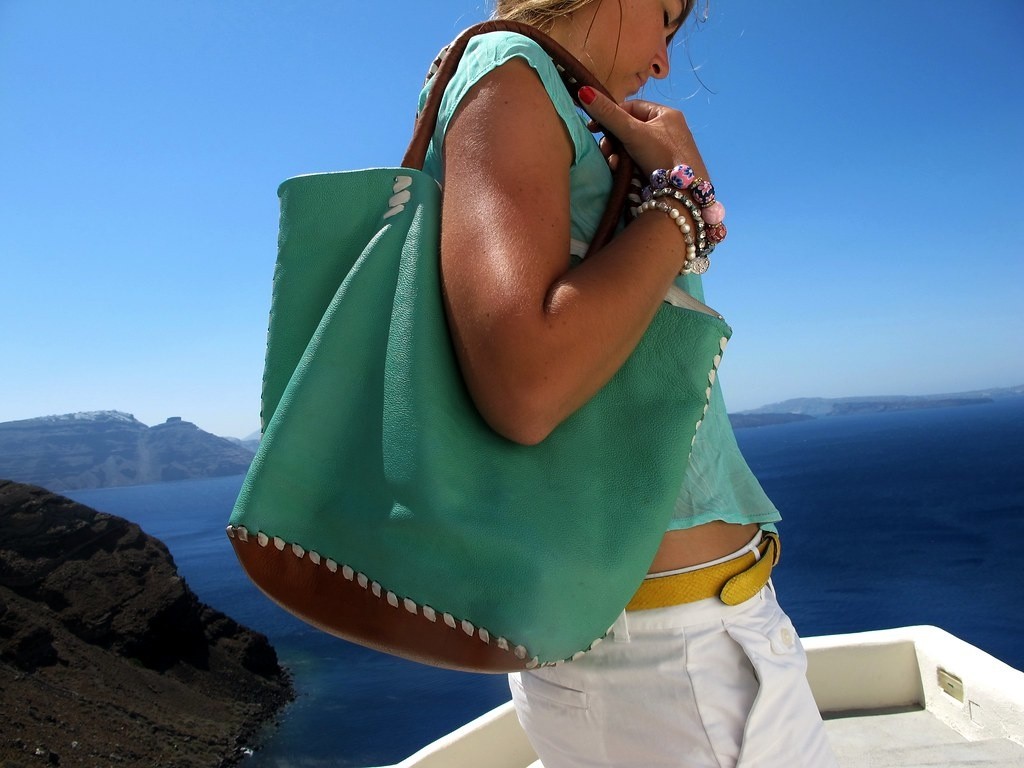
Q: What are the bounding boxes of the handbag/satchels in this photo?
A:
[224,19,735,674]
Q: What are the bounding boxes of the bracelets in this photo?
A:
[636,164,727,275]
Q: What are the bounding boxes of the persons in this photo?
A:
[414,0,842,768]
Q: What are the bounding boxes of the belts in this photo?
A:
[626,533,783,611]
